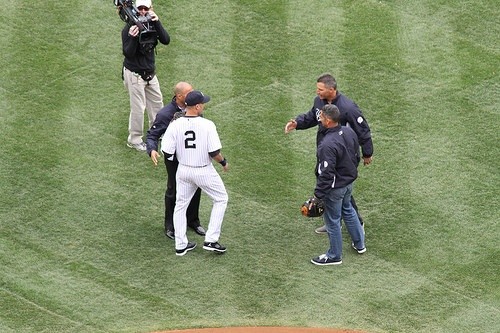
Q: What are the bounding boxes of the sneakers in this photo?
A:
[315,225,343,233]
[127,142,147,151]
[203,241,226,252]
[352,243,366,253]
[361,223,364,233]
[176,241,197,256]
[310,254,342,265]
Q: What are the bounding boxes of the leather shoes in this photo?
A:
[189,225,206,235]
[166,229,175,240]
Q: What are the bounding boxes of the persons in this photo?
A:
[122,0,171,151]
[302,105,367,267]
[283,74,374,234]
[147,83,230,255]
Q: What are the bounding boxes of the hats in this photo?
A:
[135,0,151,8]
[185,91,210,105]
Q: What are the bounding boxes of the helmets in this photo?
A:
[301,200,324,217]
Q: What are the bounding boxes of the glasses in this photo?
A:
[137,6,149,11]
